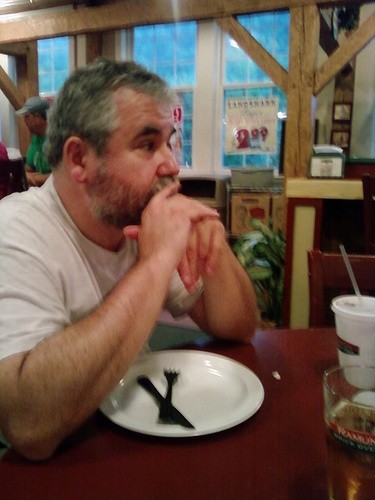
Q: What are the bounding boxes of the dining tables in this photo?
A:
[0,328,375,500]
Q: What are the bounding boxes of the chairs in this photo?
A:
[308,250,375,328]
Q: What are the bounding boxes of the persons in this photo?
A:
[14,96,52,188]
[0,60,259,460]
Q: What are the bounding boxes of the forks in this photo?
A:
[157,367,179,424]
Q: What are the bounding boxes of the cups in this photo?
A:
[331,296,375,389]
[321,365,375,465]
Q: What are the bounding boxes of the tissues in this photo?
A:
[309,143,346,178]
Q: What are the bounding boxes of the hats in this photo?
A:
[13,96,49,117]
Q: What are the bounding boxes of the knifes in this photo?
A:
[136,375,196,429]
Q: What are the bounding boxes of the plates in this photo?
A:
[98,350,264,437]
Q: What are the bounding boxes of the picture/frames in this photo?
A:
[330,130,351,151]
[332,102,352,122]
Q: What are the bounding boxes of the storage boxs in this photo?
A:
[230,168,275,189]
[231,193,286,235]
